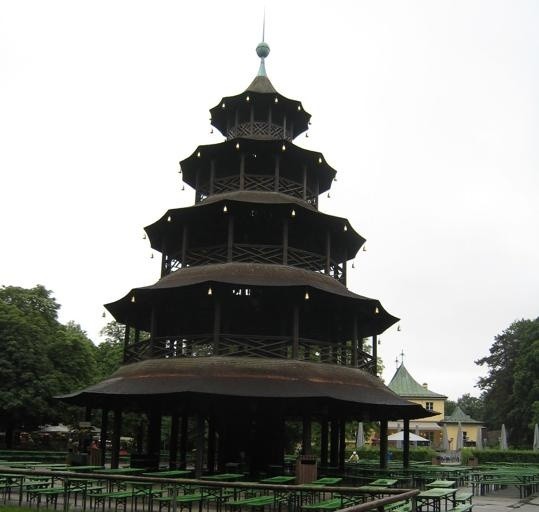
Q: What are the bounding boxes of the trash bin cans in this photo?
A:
[295,454,318,484]
[433,457,441,465]
[467,456,478,466]
[86,447,101,466]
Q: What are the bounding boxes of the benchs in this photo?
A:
[484,460,539,511]
[2,462,484,509]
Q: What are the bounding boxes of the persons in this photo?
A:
[348,451,360,463]
